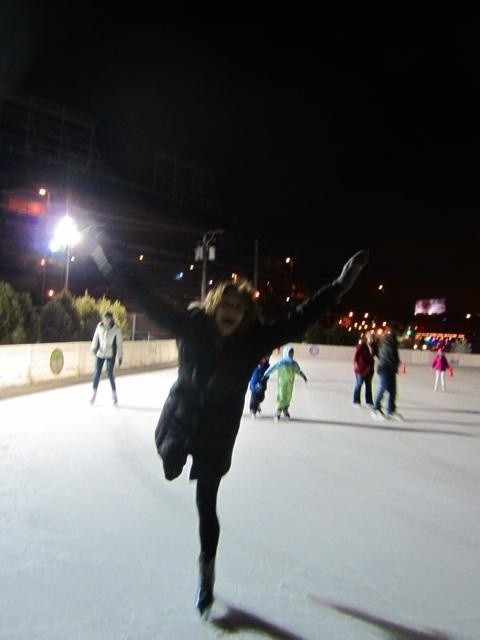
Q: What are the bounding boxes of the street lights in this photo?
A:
[57,215,76,292]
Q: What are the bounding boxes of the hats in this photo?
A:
[103,312,112,318]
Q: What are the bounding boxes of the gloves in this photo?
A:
[332,250,368,298]
[79,224,109,251]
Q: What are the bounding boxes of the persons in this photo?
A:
[431,348,449,391]
[371,323,400,413]
[66,209,373,617]
[88,311,122,404]
[353,333,374,406]
[249,353,270,415]
[261,345,307,419]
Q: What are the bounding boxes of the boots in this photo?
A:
[197,582,213,614]
[251,406,290,420]
[91,388,97,401]
[354,403,403,421]
[112,391,117,404]
